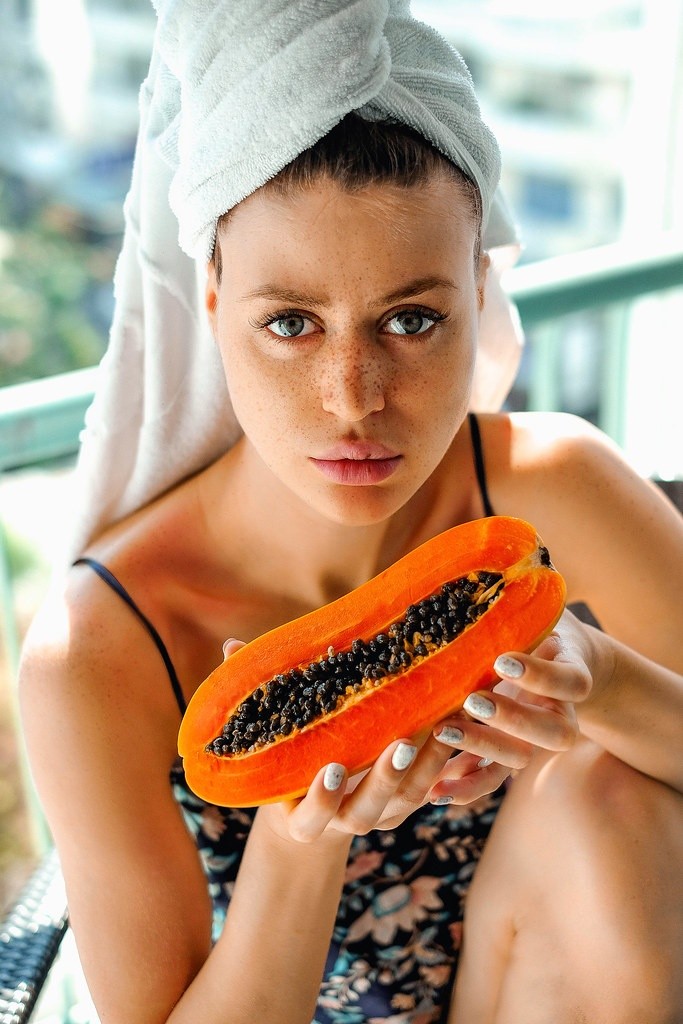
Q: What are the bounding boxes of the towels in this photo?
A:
[47,0,525,582]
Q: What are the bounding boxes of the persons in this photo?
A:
[15,1,683,1024]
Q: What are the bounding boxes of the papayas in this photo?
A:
[178,514,566,808]
